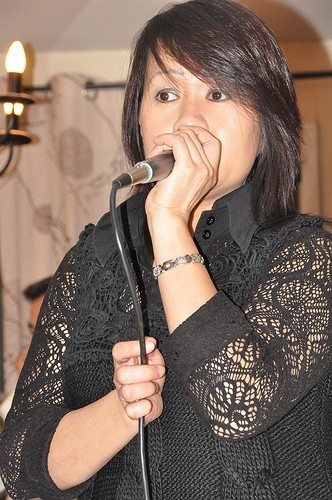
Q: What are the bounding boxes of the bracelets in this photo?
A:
[153,253,208,279]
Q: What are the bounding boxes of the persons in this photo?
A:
[0,0,332,500]
[0,276,53,420]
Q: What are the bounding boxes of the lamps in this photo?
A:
[0,40,36,146]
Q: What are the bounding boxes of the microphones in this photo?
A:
[112,151,175,189]
